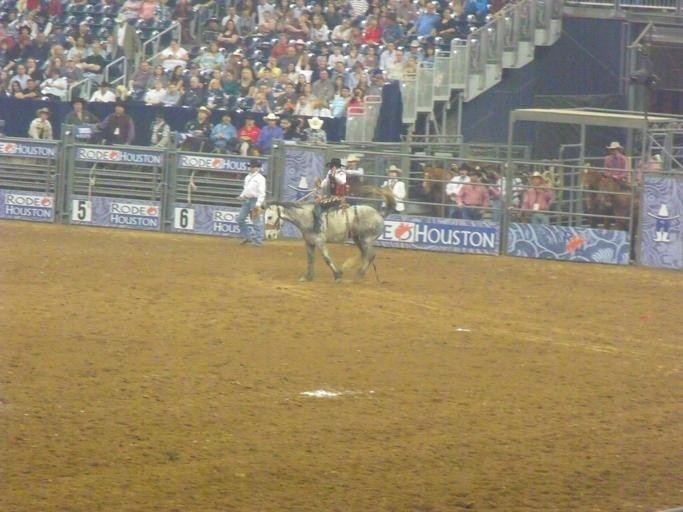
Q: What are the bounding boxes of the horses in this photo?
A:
[595,174,641,262]
[421,167,458,218]
[585,167,614,229]
[259,183,397,284]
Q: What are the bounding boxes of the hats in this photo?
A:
[326,154,359,167]
[532,171,542,177]
[459,164,480,176]
[386,165,401,172]
[245,159,261,167]
[307,117,323,129]
[37,107,51,115]
[263,113,279,122]
[607,142,622,149]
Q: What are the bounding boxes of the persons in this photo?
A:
[605,141,628,181]
[234,160,268,246]
[381,165,406,214]
[445,163,555,224]
[0,0,499,155]
[637,153,663,183]
[316,156,364,205]
